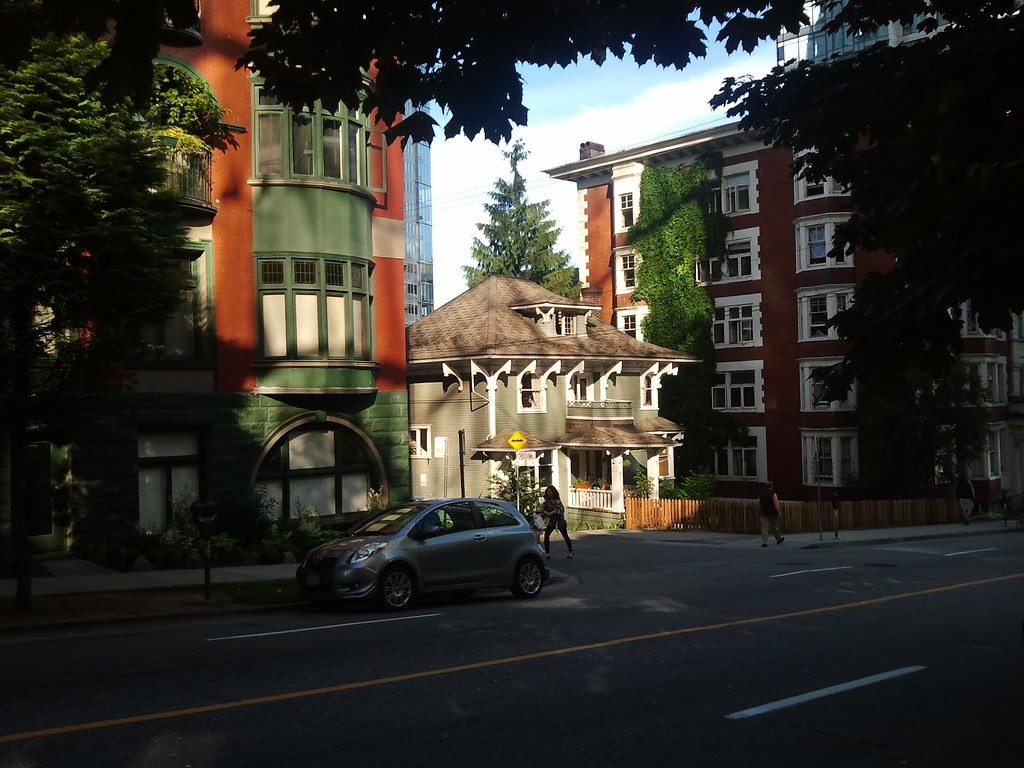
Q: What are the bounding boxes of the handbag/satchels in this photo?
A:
[533,515,547,531]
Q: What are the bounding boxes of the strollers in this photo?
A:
[524,511,548,544]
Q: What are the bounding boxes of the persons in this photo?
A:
[541,486,574,560]
[758,481,784,548]
[955,472,976,525]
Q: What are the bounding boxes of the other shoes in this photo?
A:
[964,519,970,525]
[777,537,784,544]
[762,543,768,547]
[546,556,550,560]
[567,553,574,558]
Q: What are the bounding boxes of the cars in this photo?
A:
[296,497,550,612]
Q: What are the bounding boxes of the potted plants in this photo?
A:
[132,122,207,157]
[598,478,611,490]
[573,477,589,491]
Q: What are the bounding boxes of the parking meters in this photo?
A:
[832,492,841,510]
[191,500,218,537]
